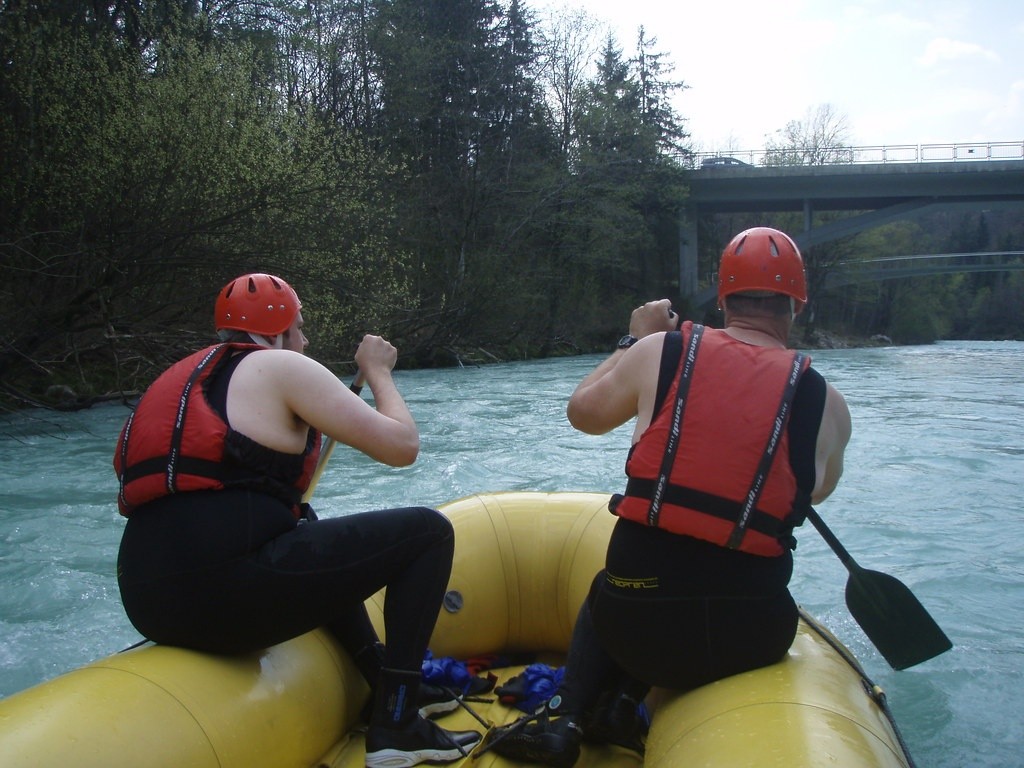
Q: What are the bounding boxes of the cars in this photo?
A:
[699,155,754,168]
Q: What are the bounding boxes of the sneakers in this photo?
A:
[417,682,464,719]
[365,715,483,768]
[490,704,584,768]
[607,705,650,757]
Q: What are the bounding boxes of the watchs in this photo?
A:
[617,334,638,348]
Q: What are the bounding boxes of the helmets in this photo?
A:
[214,273,303,335]
[717,227,808,304]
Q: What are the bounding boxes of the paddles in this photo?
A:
[300,360,366,505]
[668,307,955,673]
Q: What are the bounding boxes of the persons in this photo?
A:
[110,270,484,768]
[486,226,853,768]
